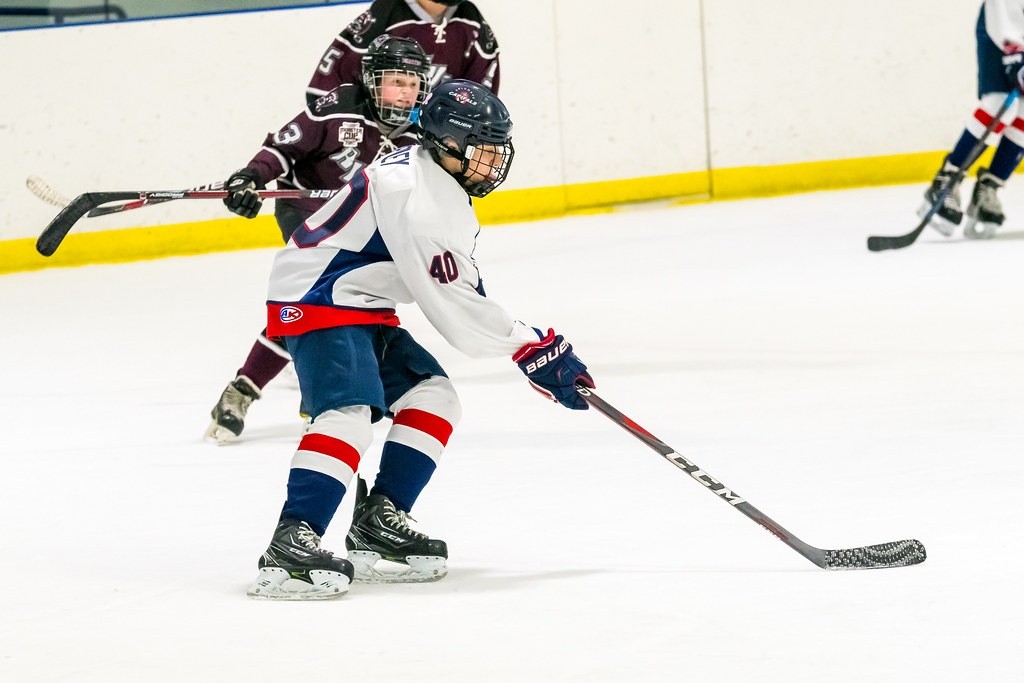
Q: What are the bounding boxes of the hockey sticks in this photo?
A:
[33,185,339,258]
[25,172,227,219]
[573,381,929,571]
[866,85,1021,253]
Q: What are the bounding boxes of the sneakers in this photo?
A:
[918,154,963,237]
[245,500,354,600]
[967,165,1005,235]
[343,472,448,583]
[207,367,261,443]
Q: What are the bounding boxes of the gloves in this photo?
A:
[223,165,265,220]
[510,326,596,411]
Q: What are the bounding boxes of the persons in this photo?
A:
[917,0,1024,238]
[202,0,501,446]
[243,80,597,600]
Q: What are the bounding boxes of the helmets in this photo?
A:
[362,34,431,135]
[418,78,515,198]
